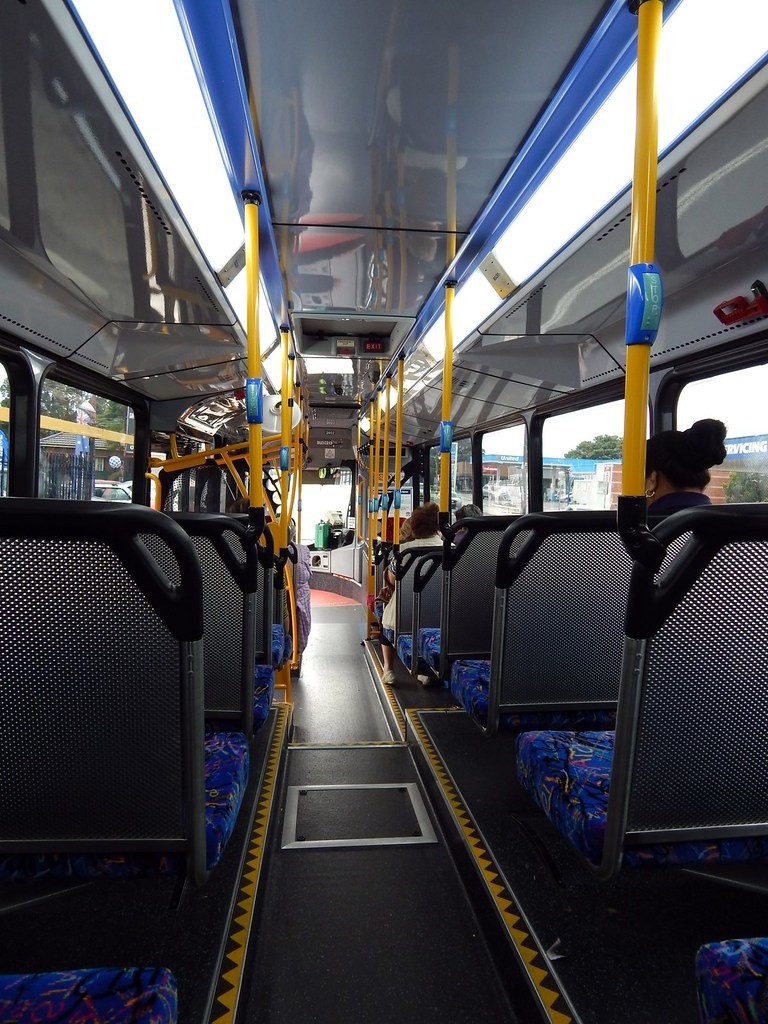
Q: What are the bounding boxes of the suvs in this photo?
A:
[435,491,462,508]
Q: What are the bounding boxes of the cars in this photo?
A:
[93,484,132,504]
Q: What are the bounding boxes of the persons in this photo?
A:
[226,498,312,654]
[643,419,727,531]
[379,502,482,687]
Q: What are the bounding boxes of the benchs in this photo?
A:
[0,496,768,1024]
